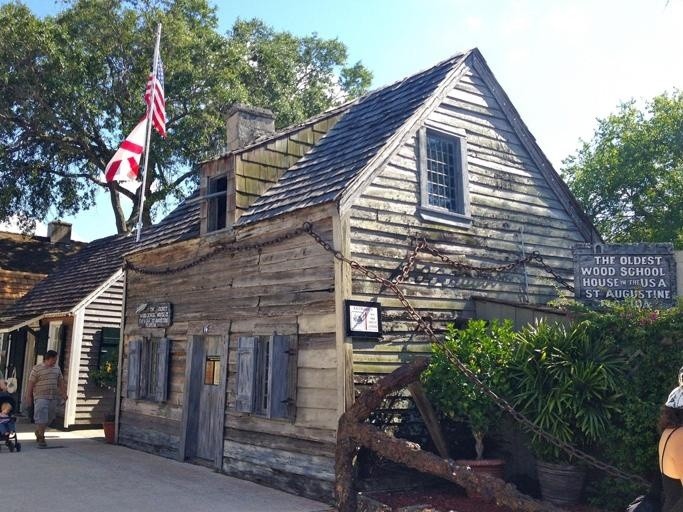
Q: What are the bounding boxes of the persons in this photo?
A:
[27,350,68,446]
[0,369,7,393]
[660,379,682,512]
[0,403,15,438]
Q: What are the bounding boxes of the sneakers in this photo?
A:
[37,435,45,446]
[35,429,45,438]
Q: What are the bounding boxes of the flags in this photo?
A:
[105,47,167,182]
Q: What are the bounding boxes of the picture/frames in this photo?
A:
[342,299,382,338]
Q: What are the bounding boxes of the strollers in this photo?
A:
[0,392,21,452]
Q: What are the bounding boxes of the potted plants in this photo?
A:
[85,354,118,444]
[497,311,626,507]
[419,310,532,502]
